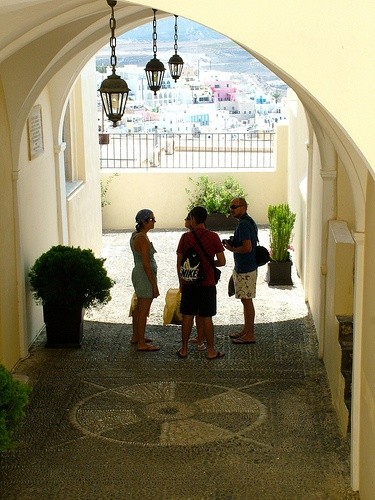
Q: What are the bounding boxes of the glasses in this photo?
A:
[230,204,245,209]
[146,218,155,222]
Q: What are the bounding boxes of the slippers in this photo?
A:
[177,348,188,358]
[130,338,152,344]
[208,351,225,359]
[198,344,206,350]
[232,337,256,344]
[137,344,160,351]
[229,331,243,338]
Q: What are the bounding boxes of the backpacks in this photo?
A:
[179,231,206,292]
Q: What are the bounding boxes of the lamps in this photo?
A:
[144,8,166,95]
[97,0,131,127]
[168,14,184,82]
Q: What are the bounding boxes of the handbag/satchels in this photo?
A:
[214,267,221,284]
[129,293,150,317]
[163,288,183,325]
[228,275,235,297]
[256,238,271,266]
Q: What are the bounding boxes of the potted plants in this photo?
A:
[29,245,115,350]
[267,205,297,285]
[185,174,249,230]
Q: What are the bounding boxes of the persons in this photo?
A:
[222,198,258,344]
[130,209,160,350]
[185,212,207,350]
[176,206,225,359]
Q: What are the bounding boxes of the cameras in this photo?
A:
[222,236,233,246]
